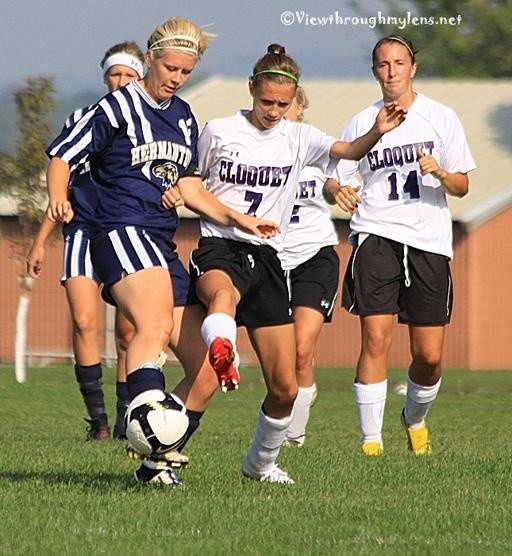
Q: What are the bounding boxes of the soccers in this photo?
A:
[123,389,190,456]
[393,379,408,396]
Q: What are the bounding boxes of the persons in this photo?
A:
[273,85,342,447]
[161,42,408,484]
[319,30,477,460]
[42,15,285,490]
[21,41,146,442]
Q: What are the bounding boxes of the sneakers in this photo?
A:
[242,455,294,485]
[124,443,189,490]
[400,407,431,456]
[209,336,241,393]
[83,413,111,440]
[360,441,383,456]
[281,433,305,448]
